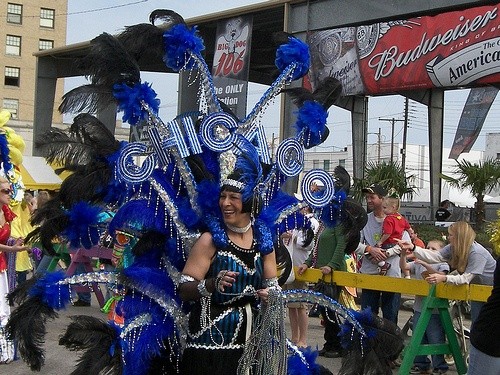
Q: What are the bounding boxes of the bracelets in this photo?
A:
[198,279,213,297]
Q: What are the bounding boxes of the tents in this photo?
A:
[14,154,76,190]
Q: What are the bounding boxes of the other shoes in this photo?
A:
[72,299,92,306]
[315,348,342,357]
[409,366,429,374]
[431,366,450,374]
[377,261,391,276]
[291,338,307,348]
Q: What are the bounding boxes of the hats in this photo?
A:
[361,183,386,196]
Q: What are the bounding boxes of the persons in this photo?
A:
[280,183,500,375]
[177,171,283,375]
[0,111,124,363]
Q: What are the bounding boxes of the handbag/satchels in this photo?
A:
[314,266,337,303]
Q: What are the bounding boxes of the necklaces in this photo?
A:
[226,220,251,232]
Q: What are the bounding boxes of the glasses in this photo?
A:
[0,188,15,195]
